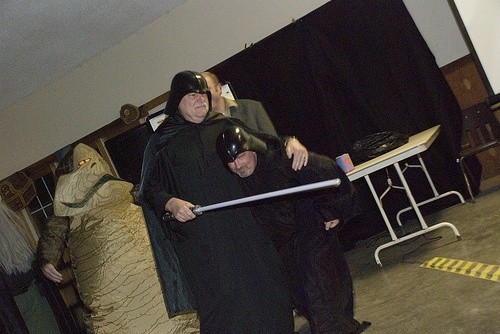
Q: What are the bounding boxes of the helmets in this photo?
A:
[215,125,268,171]
[164,71,212,115]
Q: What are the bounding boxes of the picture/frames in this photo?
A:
[145,81,239,133]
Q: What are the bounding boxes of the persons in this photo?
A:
[135,70,309,334]
[198,71,278,137]
[217,125,368,334]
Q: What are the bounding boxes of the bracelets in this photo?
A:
[282,135,297,147]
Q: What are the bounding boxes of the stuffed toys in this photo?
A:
[36,142,203,334]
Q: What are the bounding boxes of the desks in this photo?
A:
[346,124,466,268]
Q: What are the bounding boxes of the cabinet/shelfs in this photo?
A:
[31,249,86,334]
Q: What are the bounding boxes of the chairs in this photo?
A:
[460,102,500,204]
